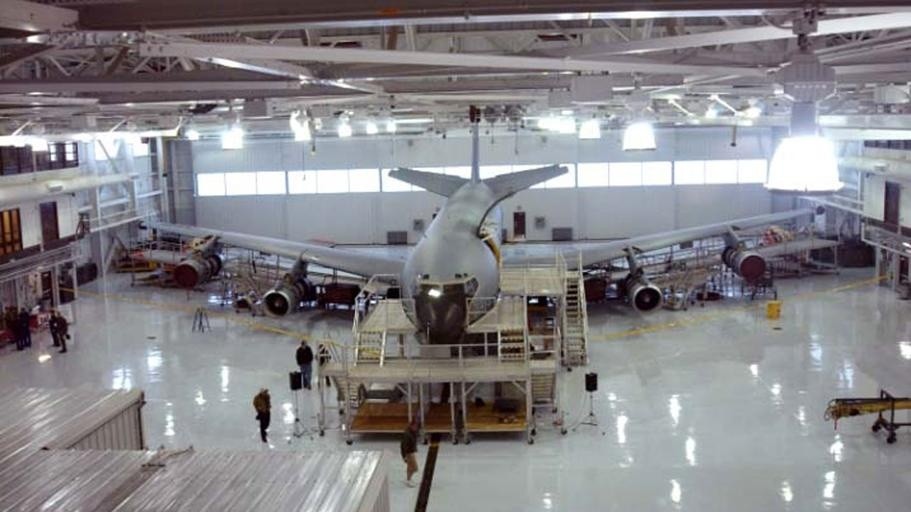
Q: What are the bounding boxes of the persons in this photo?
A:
[55,310,69,353]
[297,340,314,390]
[402,420,422,488]
[315,343,333,388]
[49,309,61,346]
[3,302,33,350]
[254,386,272,442]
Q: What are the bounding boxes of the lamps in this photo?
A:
[765,9,843,194]
[622,75,657,150]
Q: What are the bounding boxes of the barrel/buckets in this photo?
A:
[766,299,781,319]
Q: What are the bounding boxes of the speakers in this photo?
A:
[586,373,598,391]
[289,372,302,390]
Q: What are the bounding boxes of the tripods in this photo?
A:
[572,392,606,435]
[287,391,314,444]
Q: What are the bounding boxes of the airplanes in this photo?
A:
[131,161,840,344]
